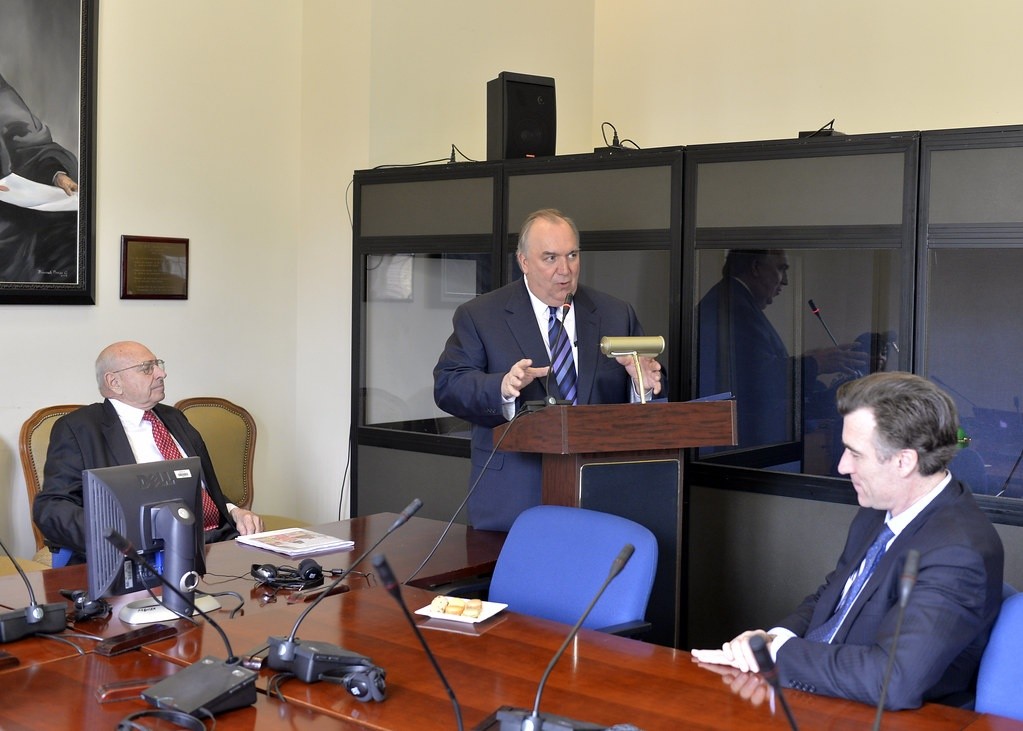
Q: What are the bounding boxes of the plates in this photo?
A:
[414,596,509,623]
[415,614,508,637]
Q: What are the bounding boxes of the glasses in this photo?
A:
[113,360,165,375]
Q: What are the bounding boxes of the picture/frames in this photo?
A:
[0,0,97,309]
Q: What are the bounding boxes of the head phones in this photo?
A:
[318,665,388,701]
[251,559,324,590]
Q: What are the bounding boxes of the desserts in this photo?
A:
[429,595,483,619]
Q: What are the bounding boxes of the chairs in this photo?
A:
[19,405,85,567]
[172,397,313,531]
[0,555,53,578]
[435,504,659,641]
[973,589,1023,720]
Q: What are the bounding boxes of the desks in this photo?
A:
[0,605,384,731]
[0,510,510,645]
[140,582,1023,731]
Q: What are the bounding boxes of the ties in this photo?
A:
[803,525,895,643]
[548,306,577,406]
[143,411,219,531]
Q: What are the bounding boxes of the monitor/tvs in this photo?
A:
[82,456,222,625]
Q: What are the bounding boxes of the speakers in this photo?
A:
[486,71,556,162]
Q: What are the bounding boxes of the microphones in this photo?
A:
[472,542,637,731]
[373,553,464,731]
[267,498,426,683]
[931,375,978,408]
[102,526,261,718]
[808,299,838,347]
[872,547,922,731]
[513,292,574,417]
[749,636,799,731]
[1014,397,1021,423]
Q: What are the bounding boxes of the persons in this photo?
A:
[692,656,776,715]
[432,208,667,532]
[830,332,889,390]
[690,371,1004,711]
[697,247,871,474]
[33,341,264,563]
